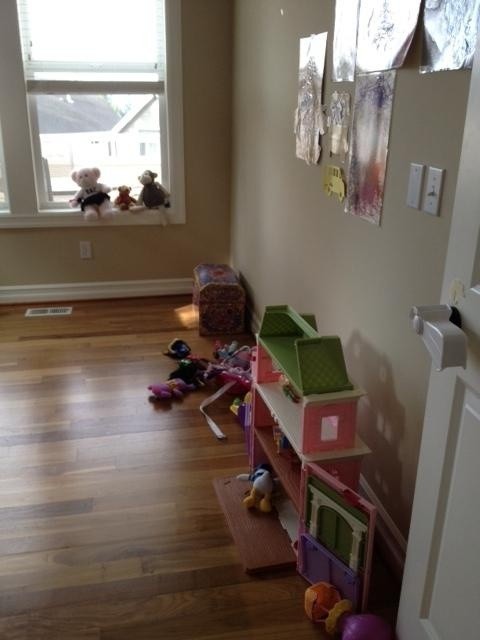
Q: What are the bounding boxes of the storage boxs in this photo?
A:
[193,263,246,336]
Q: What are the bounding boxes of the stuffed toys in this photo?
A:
[204,334,252,414]
[137,170,171,210]
[236,464,280,513]
[115,185,136,211]
[147,378,193,399]
[68,168,117,222]
